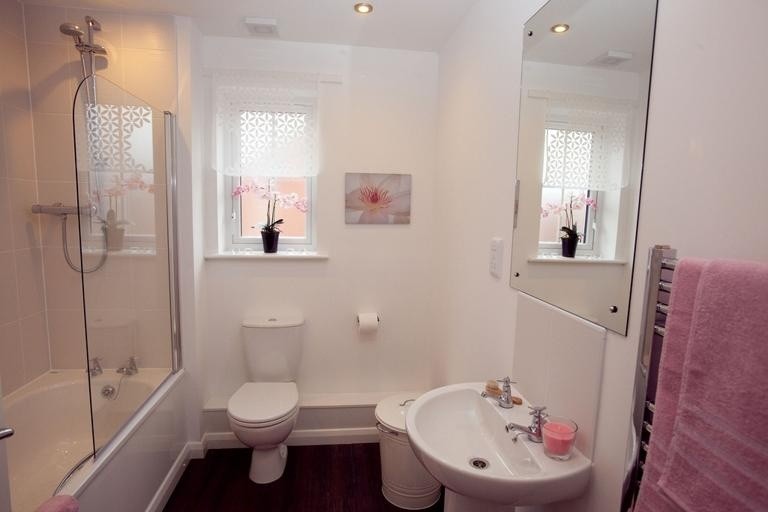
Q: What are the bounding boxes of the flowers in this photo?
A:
[87,178,132,231]
[538,185,601,240]
[232,178,311,232]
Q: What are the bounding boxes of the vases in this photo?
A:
[103,227,126,252]
[260,230,279,253]
[560,236,579,258]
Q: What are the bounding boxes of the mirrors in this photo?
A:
[510,0,661,338]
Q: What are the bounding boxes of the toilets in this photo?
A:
[226,313,305,484]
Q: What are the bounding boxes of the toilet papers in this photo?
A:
[358,312,380,333]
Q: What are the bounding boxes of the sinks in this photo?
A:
[405,379,593,503]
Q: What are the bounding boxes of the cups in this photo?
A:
[538,414,579,462]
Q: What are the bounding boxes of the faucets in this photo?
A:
[505,404,547,445]
[84,356,103,377]
[479,375,518,410]
[116,354,141,376]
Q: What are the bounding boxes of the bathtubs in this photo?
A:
[0,368,192,512]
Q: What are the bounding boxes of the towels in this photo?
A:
[631,257,768,511]
[36,494,79,512]
